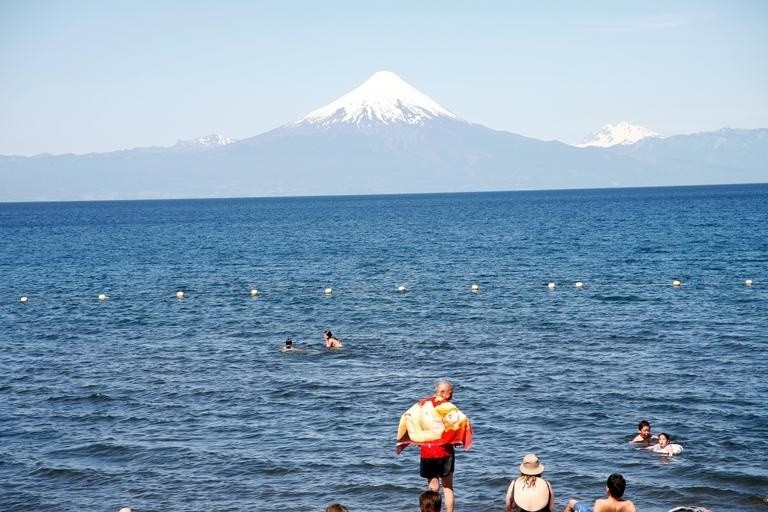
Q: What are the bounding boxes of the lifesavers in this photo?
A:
[654,444,683,455]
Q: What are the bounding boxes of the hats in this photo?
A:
[519,454,545,475]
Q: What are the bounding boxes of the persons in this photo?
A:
[280,338,296,352]
[629,421,659,445]
[633,431,674,465]
[396,381,472,512]
[504,454,556,512]
[321,330,345,350]
[418,491,444,512]
[563,473,636,512]
